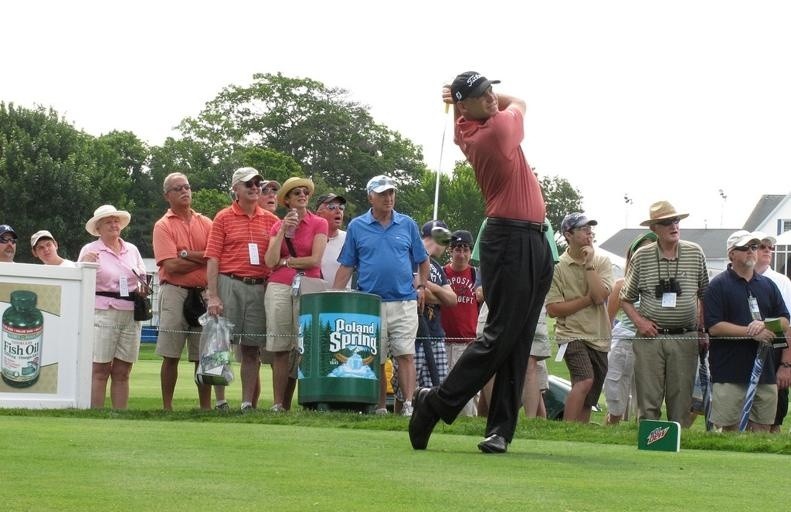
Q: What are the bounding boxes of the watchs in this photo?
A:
[181,249,187,258]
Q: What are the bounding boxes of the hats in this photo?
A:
[630,230,657,255]
[30,230,55,247]
[422,220,474,249]
[727,230,761,251]
[316,192,346,210]
[0,224,18,239]
[260,179,281,195]
[450,71,501,102]
[751,230,777,244]
[277,177,314,208]
[367,175,399,194]
[85,205,131,237]
[561,213,598,232]
[231,167,263,187]
[639,201,689,226]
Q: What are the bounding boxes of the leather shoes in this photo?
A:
[409,386,440,449]
[477,434,508,453]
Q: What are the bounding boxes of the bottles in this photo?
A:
[285,208,298,238]
[2,290,43,388]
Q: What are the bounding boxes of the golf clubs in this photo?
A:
[431,103,452,246]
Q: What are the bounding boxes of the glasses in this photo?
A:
[734,244,759,251]
[1,237,17,244]
[291,189,310,195]
[760,243,772,250]
[246,180,261,187]
[328,202,345,210]
[167,183,190,193]
[655,217,680,225]
[262,188,278,194]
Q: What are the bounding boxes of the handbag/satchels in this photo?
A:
[182,290,208,326]
[292,275,328,330]
[133,298,153,321]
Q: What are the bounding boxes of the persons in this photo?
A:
[153,173,215,412]
[199,166,281,412]
[316,174,790,430]
[0,224,18,262]
[29,228,75,267]
[235,179,281,409]
[77,204,150,411]
[406,70,555,454]
[265,177,329,413]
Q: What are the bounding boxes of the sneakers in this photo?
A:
[213,401,414,417]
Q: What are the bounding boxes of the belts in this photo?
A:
[163,280,205,293]
[657,326,696,334]
[487,218,549,233]
[221,272,267,285]
